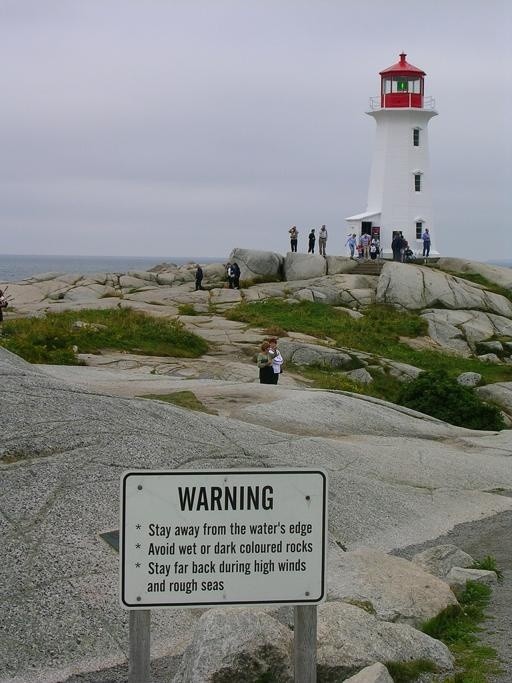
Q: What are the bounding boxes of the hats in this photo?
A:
[261,343,271,350]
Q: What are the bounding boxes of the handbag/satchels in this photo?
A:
[280,365,283,374]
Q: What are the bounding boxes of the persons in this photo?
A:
[268,339,284,384]
[345,230,408,262]
[194,264,203,291]
[288,225,299,252]
[233,263,241,290]
[422,228,431,257]
[307,229,315,254]
[319,225,328,257]
[227,263,234,289]
[257,340,277,384]
